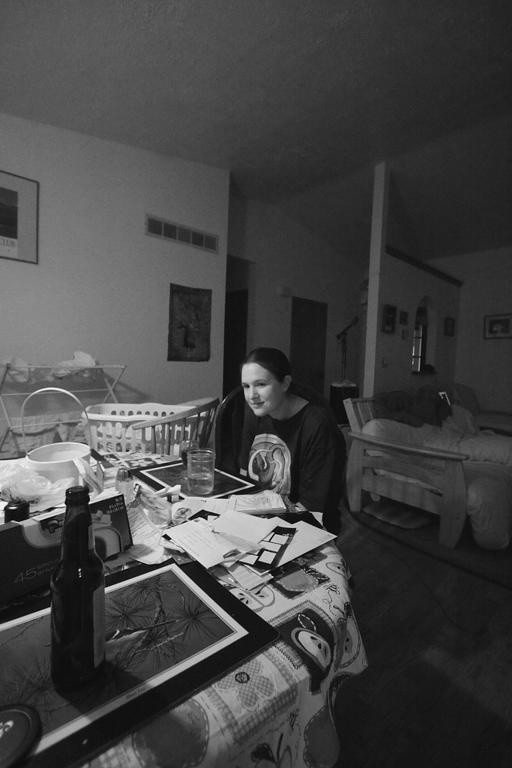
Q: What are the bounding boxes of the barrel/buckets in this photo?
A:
[19,386,93,488]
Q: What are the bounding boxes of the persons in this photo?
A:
[210,344,347,555]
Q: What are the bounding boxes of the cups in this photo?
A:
[179,438,217,497]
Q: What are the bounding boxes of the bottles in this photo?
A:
[49,483,107,700]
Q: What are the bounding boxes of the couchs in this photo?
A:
[341,380,511,549]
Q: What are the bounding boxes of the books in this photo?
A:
[172,485,338,592]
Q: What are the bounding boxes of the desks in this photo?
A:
[1,453,348,767]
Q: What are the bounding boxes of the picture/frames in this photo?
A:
[483,313,512,340]
[0,169,40,264]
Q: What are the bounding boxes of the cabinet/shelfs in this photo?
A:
[0,359,128,458]
